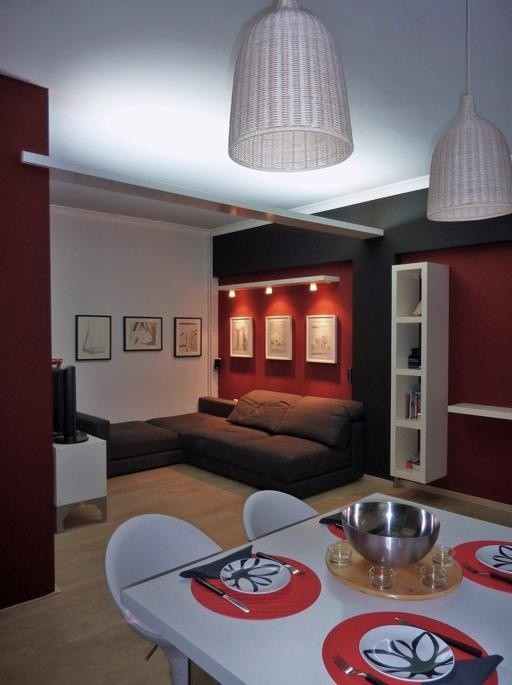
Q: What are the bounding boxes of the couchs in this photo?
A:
[143,389,369,500]
[74,408,185,479]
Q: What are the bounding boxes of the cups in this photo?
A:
[432,544,455,569]
[327,542,354,569]
[419,564,449,589]
[367,565,395,590]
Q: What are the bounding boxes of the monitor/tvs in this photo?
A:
[53,366,88,444]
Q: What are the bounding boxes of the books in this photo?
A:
[405,392,420,419]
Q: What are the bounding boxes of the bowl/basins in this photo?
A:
[339,499,442,570]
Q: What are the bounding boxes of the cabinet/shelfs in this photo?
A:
[54,433,108,534]
[389,260,449,485]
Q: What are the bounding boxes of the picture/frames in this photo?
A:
[263,314,294,362]
[171,315,204,360]
[121,314,164,353]
[227,316,255,360]
[304,312,339,366]
[73,313,114,362]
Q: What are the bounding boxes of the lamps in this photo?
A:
[418,0,512,229]
[226,1,354,174]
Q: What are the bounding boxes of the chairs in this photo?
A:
[103,509,230,685]
[241,485,325,543]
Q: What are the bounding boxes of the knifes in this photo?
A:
[192,576,249,615]
[394,614,484,660]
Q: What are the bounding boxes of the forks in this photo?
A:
[330,654,383,684]
[460,559,510,584]
[255,550,303,578]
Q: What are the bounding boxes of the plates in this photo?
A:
[472,545,511,575]
[357,626,455,683]
[219,558,292,595]
